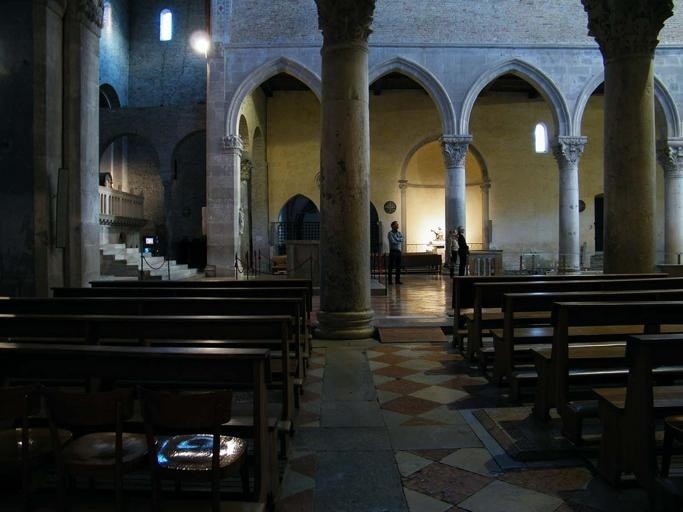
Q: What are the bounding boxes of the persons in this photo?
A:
[448,225,471,277]
[387,221,405,285]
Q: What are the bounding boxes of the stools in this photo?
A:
[139,387,251,511]
[53,388,156,511]
[0,382,73,511]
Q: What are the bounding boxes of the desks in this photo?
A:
[469,250,502,276]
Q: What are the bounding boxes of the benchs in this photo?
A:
[590,334,682,504]
[0,278,313,501]
[452,273,678,405]
[529,299,683,456]
[372,252,441,278]
